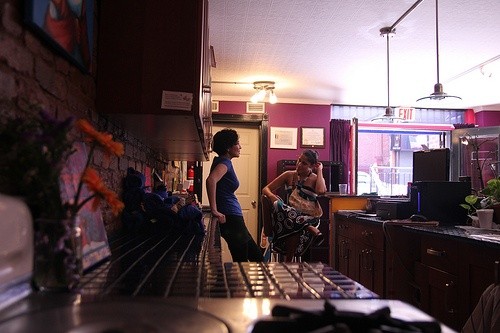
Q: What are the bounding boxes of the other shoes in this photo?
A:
[313,232,327,248]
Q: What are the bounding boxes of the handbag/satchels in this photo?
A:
[289,187,323,218]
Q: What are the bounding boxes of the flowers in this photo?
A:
[0,97,126,288]
[460,127,500,218]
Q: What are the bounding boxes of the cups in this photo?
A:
[476,209,494,229]
[339,184,347,196]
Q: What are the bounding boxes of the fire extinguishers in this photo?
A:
[187,163,196,191]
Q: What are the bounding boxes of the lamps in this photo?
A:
[370,27,404,122]
[250,80,278,106]
[415,0,463,103]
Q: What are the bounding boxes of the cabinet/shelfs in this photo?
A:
[336,209,500,333]
[277,159,344,263]
[96,0,218,161]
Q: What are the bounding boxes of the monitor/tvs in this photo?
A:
[412,148,450,183]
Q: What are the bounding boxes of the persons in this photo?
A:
[206,129,265,262]
[262,149,327,262]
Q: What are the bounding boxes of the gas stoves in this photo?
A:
[0,289,457,333]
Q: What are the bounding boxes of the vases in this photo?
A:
[29,216,83,297]
[476,209,494,229]
